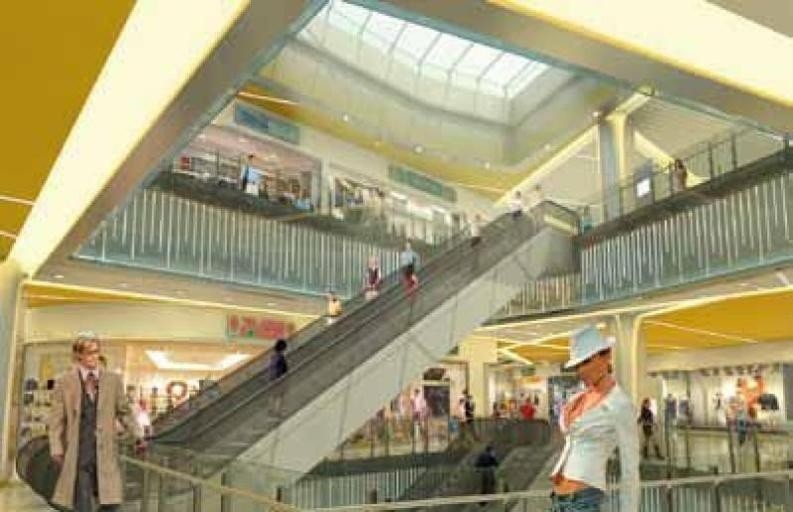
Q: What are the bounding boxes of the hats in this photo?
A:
[563,327,617,369]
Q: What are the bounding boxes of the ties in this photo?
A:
[86,371,96,403]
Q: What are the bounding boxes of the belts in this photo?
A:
[550,488,600,502]
